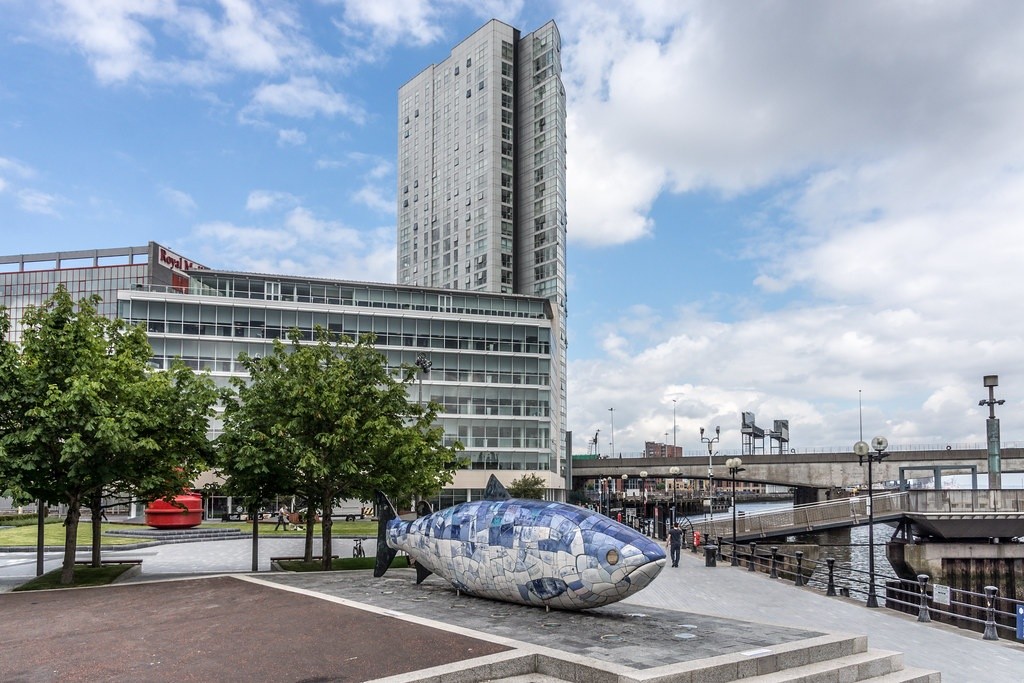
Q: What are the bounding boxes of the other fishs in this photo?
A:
[374,473,666,610]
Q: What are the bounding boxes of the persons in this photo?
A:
[236,505,243,520]
[666,523,683,567]
[275,504,290,531]
[101,509,108,521]
[259,507,266,519]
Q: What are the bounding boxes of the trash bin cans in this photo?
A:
[703,545,719,567]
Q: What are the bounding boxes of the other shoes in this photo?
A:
[676,565,678,567]
[672,563,675,567]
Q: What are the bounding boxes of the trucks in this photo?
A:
[298,490,364,522]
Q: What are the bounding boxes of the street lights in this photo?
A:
[665,433,670,458]
[726,457,747,565]
[979,375,1007,491]
[607,477,613,520]
[669,466,682,525]
[672,399,678,458]
[602,477,607,516]
[858,389,864,442]
[622,474,629,525]
[699,425,721,519]
[639,470,648,536]
[609,407,616,458]
[853,435,891,608]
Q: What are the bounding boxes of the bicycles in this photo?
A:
[353,536,366,558]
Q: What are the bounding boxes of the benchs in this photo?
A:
[62,560,144,565]
[270,555,340,562]
